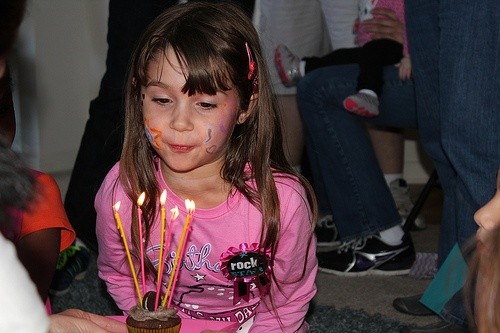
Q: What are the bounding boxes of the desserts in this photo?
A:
[125,291,181,333]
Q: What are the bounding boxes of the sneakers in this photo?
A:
[274,43,304,88]
[315,233,417,277]
[49,238,92,298]
[343,92,379,117]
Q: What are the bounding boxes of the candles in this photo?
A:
[111,189,196,311]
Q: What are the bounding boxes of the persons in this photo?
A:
[249,1,500,333]
[0,137,76,317]
[0,235,131,333]
[93,1,318,333]
[0,0,29,151]
[45,0,256,296]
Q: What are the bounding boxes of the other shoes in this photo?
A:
[393,292,438,317]
[313,178,423,248]
